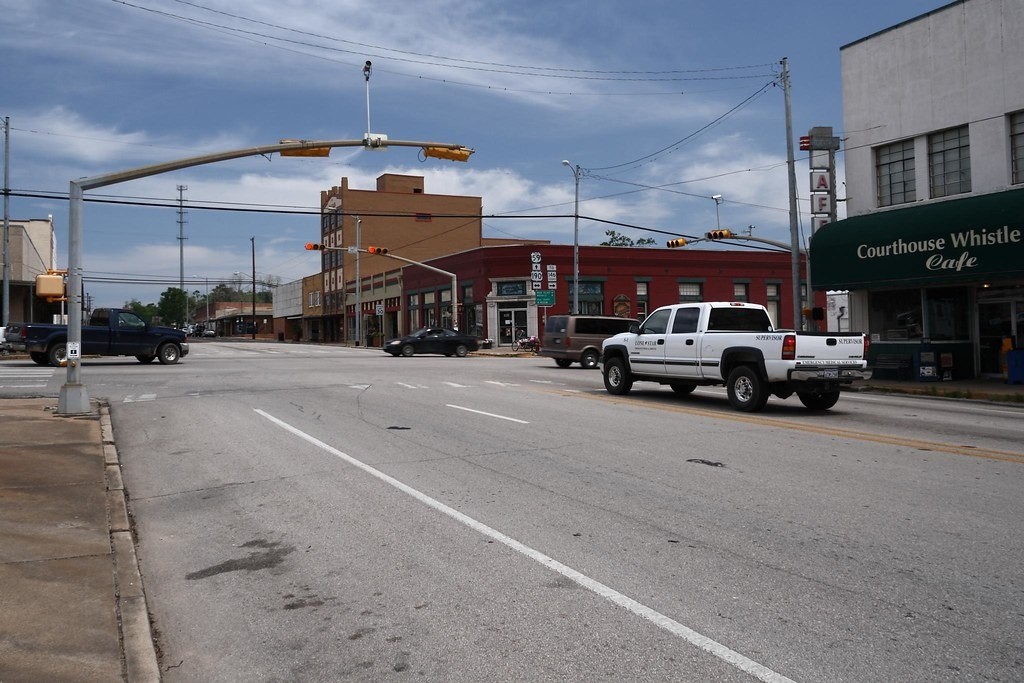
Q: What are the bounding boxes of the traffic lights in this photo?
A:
[667,238,686,248]
[368,246,389,255]
[708,230,730,239]
[305,243,326,250]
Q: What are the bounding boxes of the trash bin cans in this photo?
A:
[483,340,492,349]
[278,332,284,341]
[1006,350,1024,385]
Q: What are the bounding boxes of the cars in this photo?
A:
[182,325,203,337]
[384,327,481,357]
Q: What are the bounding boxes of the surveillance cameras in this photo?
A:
[712,195,722,199]
[362,60,372,71]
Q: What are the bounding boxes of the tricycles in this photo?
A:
[512,329,539,352]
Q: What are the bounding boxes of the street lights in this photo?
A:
[193,275,209,322]
[234,271,255,339]
[562,160,579,314]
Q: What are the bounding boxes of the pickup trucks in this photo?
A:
[0,308,189,364]
[602,301,873,412]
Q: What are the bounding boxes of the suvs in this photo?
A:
[541,313,642,368]
